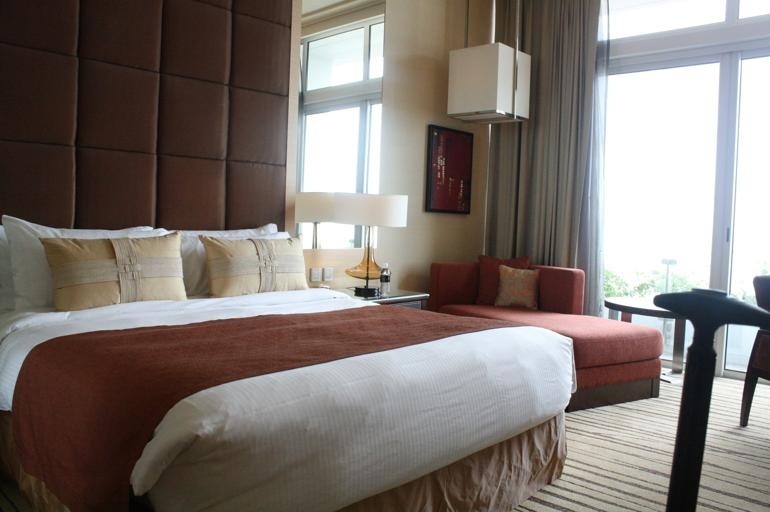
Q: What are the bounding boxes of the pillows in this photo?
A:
[1,212,166,311]
[476,255,534,306]
[0,224,14,313]
[174,221,289,301]
[206,229,292,242]
[36,228,191,315]
[198,231,311,299]
[494,264,545,310]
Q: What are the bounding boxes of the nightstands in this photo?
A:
[328,283,429,311]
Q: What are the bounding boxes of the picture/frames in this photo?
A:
[424,124,477,216]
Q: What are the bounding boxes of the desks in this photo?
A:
[604,295,692,373]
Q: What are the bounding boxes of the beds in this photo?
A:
[0,210,577,511]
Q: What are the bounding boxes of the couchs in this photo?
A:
[428,254,662,412]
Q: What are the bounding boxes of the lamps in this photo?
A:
[447,0,532,125]
[294,192,333,249]
[327,192,409,296]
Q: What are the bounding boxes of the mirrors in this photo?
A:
[293,1,386,251]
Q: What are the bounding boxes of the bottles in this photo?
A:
[380,262,391,298]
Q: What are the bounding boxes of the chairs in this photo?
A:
[739,272,770,427]
